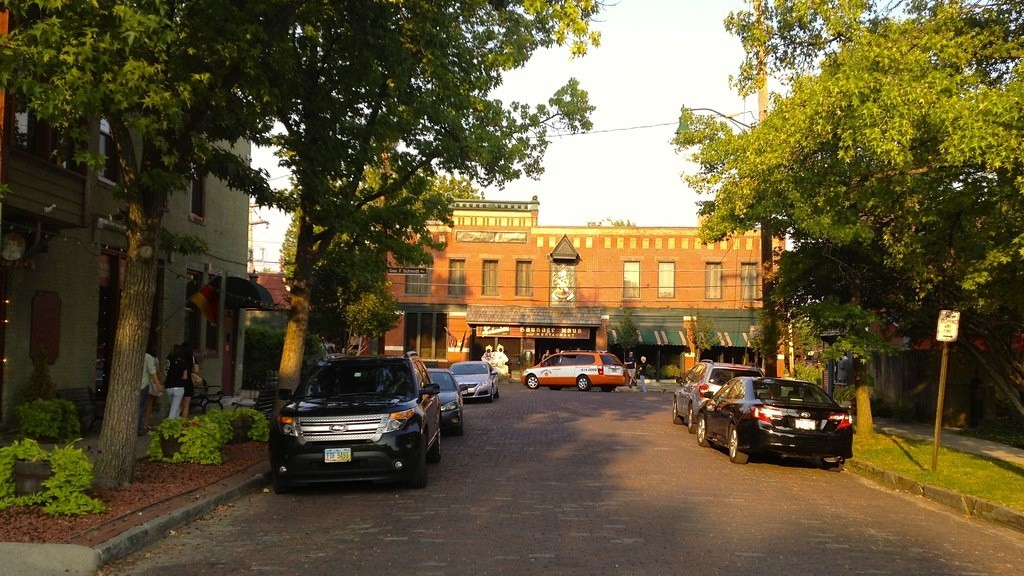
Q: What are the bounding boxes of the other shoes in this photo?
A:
[635,381,637,386]
[628,384,632,388]
[144,424,151,430]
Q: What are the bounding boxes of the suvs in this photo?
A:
[266,349,444,493]
[672,358,772,435]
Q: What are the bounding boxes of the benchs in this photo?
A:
[192,379,225,414]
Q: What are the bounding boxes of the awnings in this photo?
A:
[226,275,276,312]
[694,331,755,348]
[606,327,690,348]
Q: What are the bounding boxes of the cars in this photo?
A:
[449,360,501,403]
[391,366,470,438]
[521,351,630,392]
[694,376,853,473]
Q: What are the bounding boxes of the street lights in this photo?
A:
[676,106,777,376]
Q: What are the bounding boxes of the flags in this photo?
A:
[188,272,223,327]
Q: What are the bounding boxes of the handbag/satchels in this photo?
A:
[152,397,161,412]
[149,374,165,397]
[191,357,202,385]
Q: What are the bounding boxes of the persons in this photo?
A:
[541,348,560,366]
[638,356,647,392]
[137,340,199,435]
[624,351,637,388]
[576,348,584,360]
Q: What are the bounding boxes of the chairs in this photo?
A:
[788,390,799,399]
